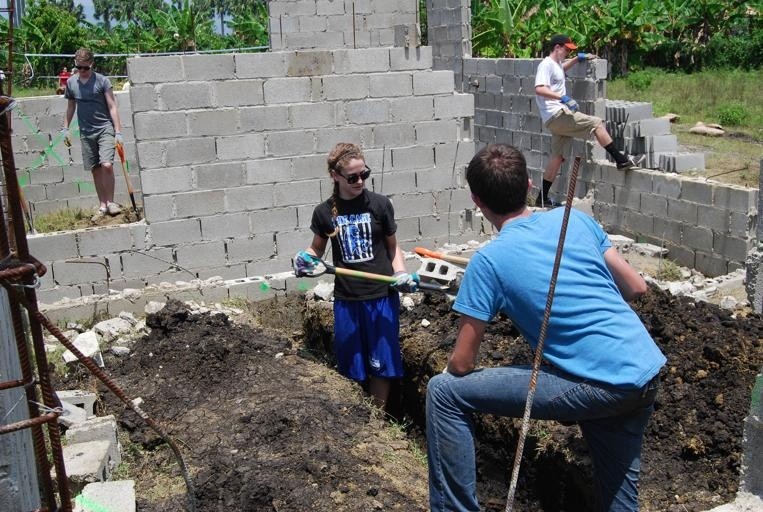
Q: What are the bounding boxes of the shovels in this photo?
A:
[300,253,465,297]
[117,144,142,222]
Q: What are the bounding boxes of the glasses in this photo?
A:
[335,165,371,184]
[75,60,93,71]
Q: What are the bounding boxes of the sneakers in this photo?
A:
[91,202,121,222]
[617,146,646,171]
[536,198,561,208]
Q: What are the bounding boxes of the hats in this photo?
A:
[550,34,577,50]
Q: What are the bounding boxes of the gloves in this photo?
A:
[293,249,319,278]
[390,271,422,293]
[578,52,592,61]
[115,132,123,147]
[560,95,578,110]
[64,128,72,147]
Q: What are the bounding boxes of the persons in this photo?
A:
[63,49,123,223]
[293,143,420,414]
[59,67,78,94]
[425,144,667,512]
[534,35,645,208]
